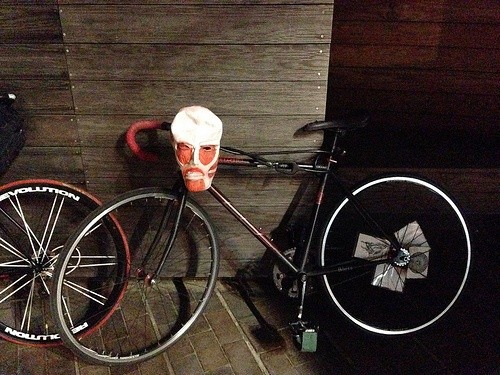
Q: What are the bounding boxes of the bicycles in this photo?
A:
[0,76,131,347]
[49,115,478,367]
